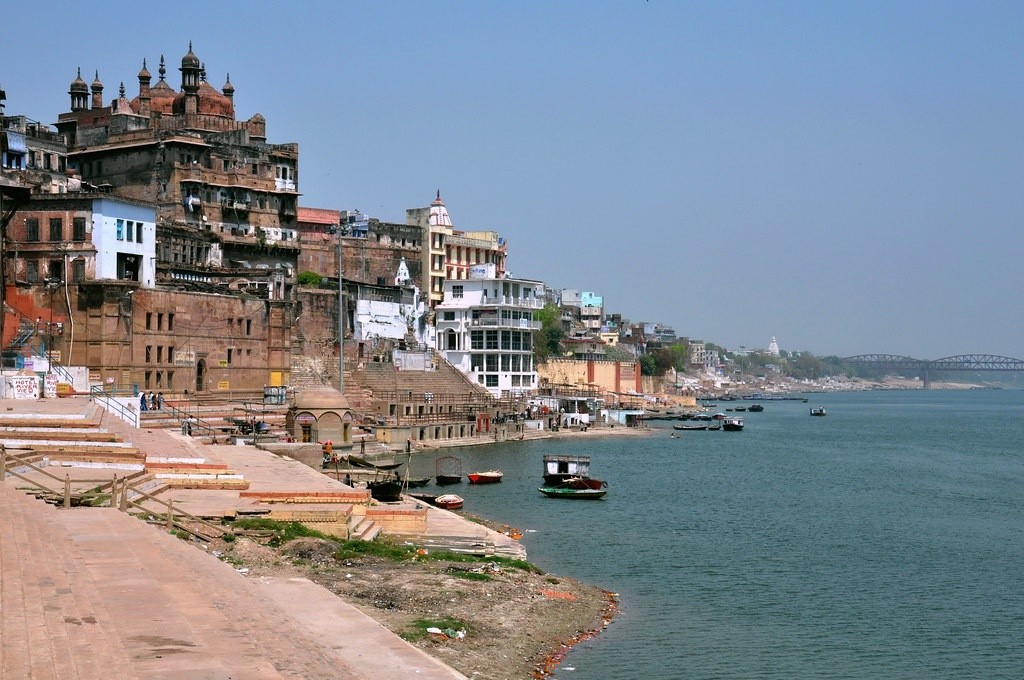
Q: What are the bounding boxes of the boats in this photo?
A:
[735,406,747,411]
[393,475,435,488]
[672,411,727,430]
[435,493,464,509]
[536,454,610,499]
[347,454,404,470]
[748,404,764,412]
[468,469,504,484]
[726,408,733,412]
[435,456,463,484]
[407,493,437,504]
[808,403,827,416]
[703,403,717,407]
[368,479,403,501]
[723,416,745,430]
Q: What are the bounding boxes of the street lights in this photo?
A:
[329,223,352,395]
[740,344,747,390]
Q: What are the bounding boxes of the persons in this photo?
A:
[360,437,366,453]
[343,473,357,488]
[138,391,163,410]
[525,405,566,432]
[188,415,193,436]
[251,416,267,434]
[393,471,400,484]
